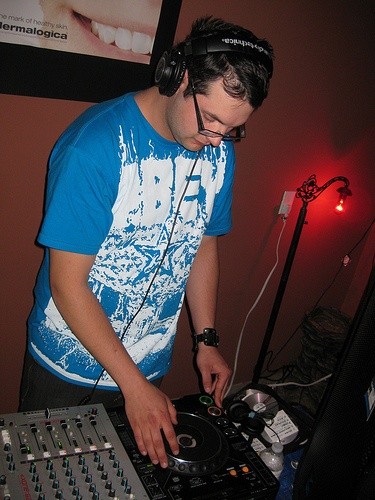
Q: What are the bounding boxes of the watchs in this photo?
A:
[193,328,220,348]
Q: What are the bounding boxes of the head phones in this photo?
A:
[153,36,272,97]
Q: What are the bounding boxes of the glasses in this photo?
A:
[188,84,247,143]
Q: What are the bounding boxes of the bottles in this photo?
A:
[258,443,284,480]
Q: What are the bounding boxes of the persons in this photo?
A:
[18,14,278,470]
[41,0,161,65]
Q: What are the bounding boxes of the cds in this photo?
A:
[245,392,280,420]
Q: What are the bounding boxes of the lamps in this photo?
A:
[252,175,352,384]
